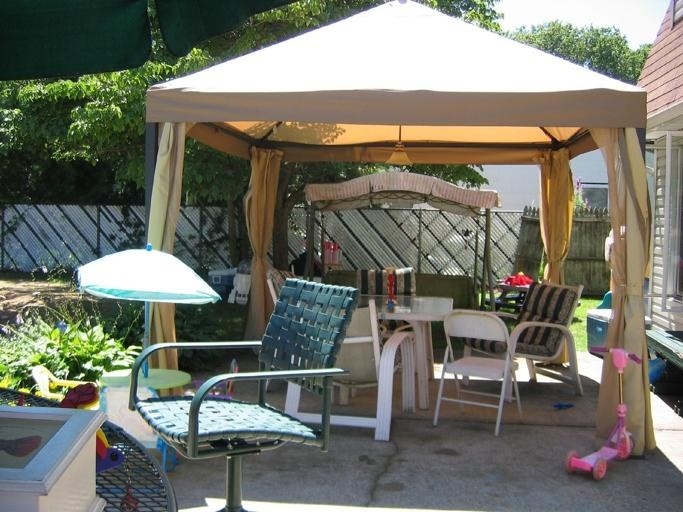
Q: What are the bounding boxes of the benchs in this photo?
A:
[642,329,683,370]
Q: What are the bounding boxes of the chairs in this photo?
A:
[266,266,416,442]
[410,296,454,314]
[430,309,523,436]
[32,364,100,409]
[461,281,584,402]
[129,275,361,512]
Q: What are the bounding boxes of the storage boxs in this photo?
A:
[207,267,236,293]
[586,308,652,356]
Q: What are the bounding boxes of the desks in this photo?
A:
[0,386,178,512]
[101,367,191,396]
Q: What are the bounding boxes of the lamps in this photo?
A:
[384,125,413,166]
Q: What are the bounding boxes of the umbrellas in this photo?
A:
[77,245,221,378]
